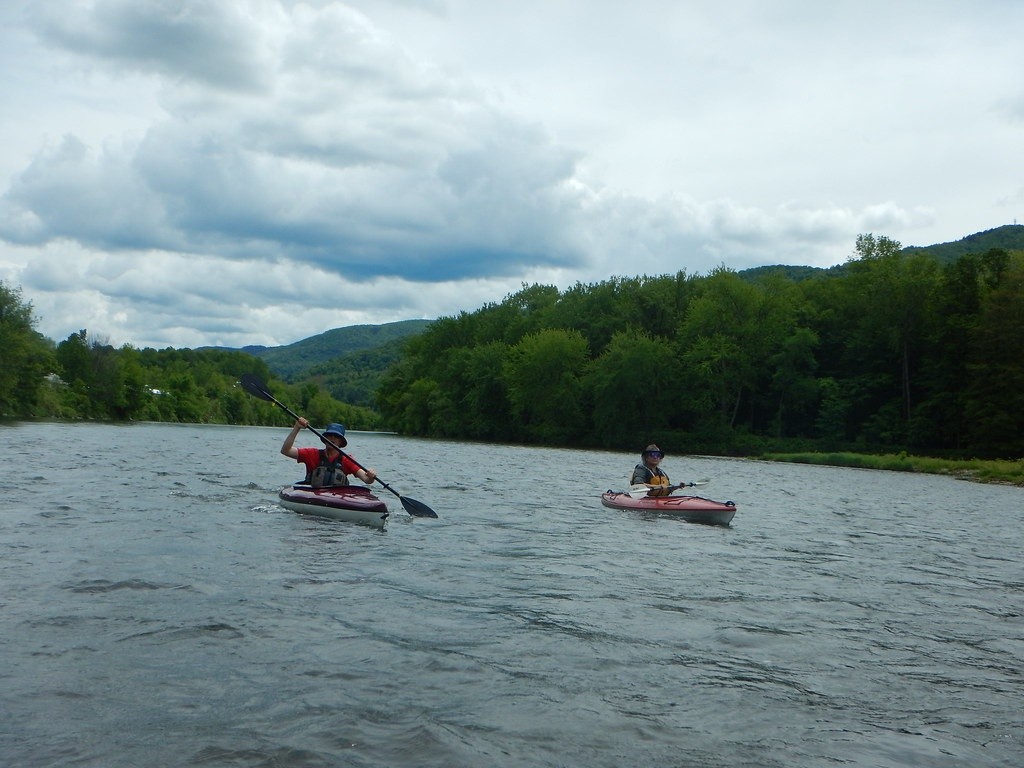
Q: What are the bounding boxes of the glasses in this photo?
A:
[642,451,664,459]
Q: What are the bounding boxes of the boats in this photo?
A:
[600,488,738,526]
[279,479,389,528]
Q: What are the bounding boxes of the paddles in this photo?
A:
[241,373,438,518]
[629,478,711,500]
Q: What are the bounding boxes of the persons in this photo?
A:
[630,444,685,496]
[280,416,376,487]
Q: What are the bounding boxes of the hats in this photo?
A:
[320,423,347,448]
[641,444,664,458]
